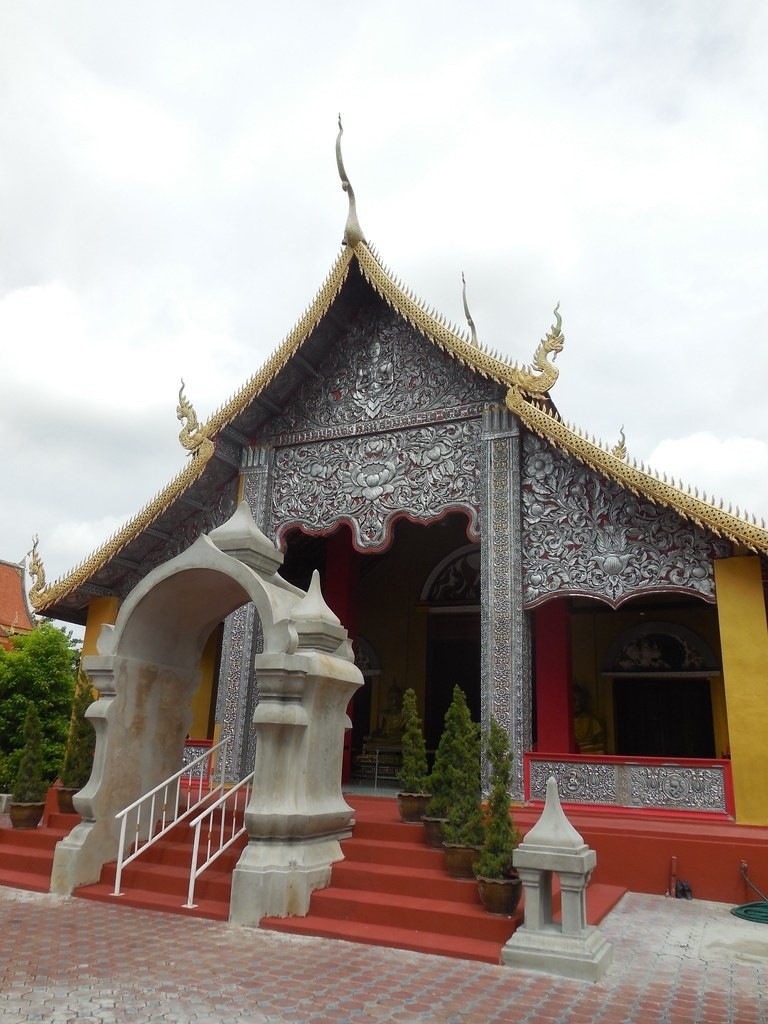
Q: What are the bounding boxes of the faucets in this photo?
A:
[739,859,748,873]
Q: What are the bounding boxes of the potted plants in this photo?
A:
[56,664,96,815]
[420,684,487,880]
[472,715,522,916]
[9,701,50,829]
[396,689,432,825]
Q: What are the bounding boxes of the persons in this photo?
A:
[573,684,605,753]
[363,685,407,746]
